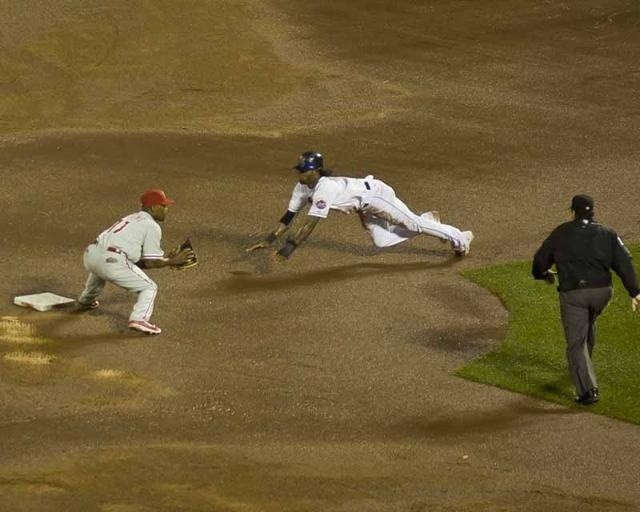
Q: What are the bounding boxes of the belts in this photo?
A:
[95,240,121,254]
[570,281,611,290]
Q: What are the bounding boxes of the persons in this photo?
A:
[79,189,195,336]
[245,150,474,263]
[533,194,640,405]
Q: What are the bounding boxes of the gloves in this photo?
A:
[544,269,557,285]
[631,293,640,313]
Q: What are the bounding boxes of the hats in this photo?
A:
[570,194,594,210]
[140,189,175,209]
[293,151,323,170]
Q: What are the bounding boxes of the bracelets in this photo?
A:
[266,233,277,244]
[284,239,296,253]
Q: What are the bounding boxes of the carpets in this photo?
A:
[451,241,640,428]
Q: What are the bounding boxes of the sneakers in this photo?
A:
[574,388,599,406]
[78,299,99,310]
[429,210,446,244]
[128,320,161,336]
[454,230,474,259]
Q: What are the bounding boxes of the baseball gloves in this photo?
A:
[169,238,198,269]
